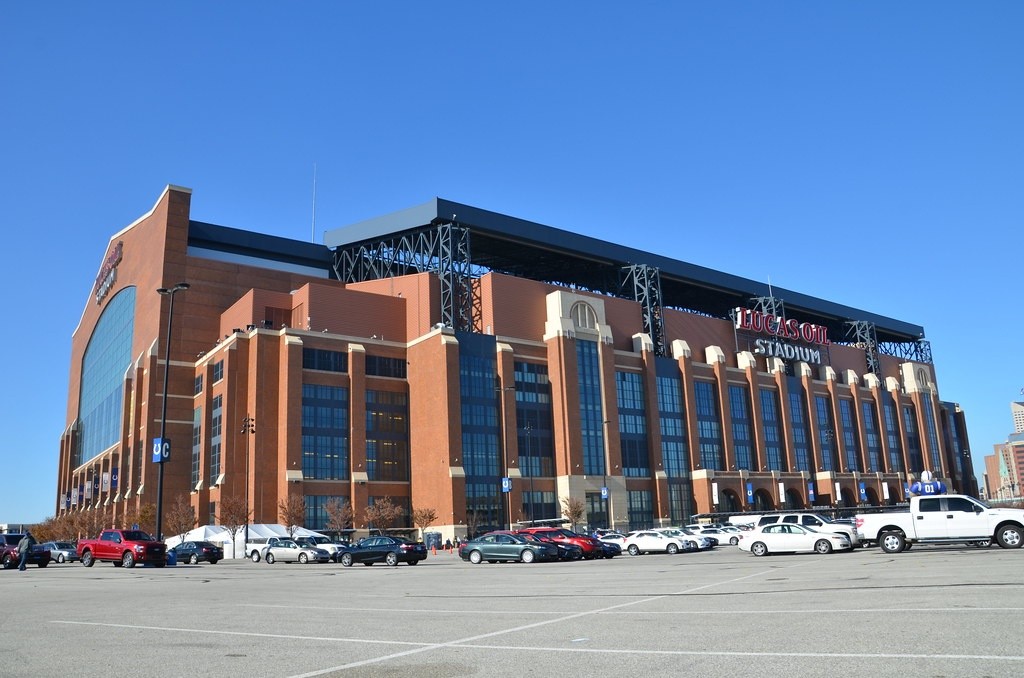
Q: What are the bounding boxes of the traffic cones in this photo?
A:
[443,544,447,552]
[433,547,437,555]
[450,545,453,554]
[432,545,434,553]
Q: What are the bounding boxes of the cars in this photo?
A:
[335,536,428,567]
[737,523,852,557]
[456,522,754,564]
[261,539,331,565]
[833,517,857,536]
[171,541,220,566]
[42,542,80,563]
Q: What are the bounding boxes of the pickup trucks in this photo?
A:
[245,537,295,562]
[855,494,1024,555]
[77,530,167,568]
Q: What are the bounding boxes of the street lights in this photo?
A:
[992,484,1013,505]
[495,385,515,531]
[524,423,535,527]
[825,429,840,514]
[601,419,611,526]
[241,413,256,559]
[151,283,190,540]
[962,450,974,494]
[1016,482,1023,504]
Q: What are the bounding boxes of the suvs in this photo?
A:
[294,536,347,558]
[0,533,51,568]
[755,512,862,550]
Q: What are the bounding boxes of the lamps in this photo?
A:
[322,329,328,333]
[370,335,376,339]
[282,324,288,328]
[261,320,273,329]
[197,320,256,357]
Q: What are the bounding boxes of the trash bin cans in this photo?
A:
[167,549,177,565]
